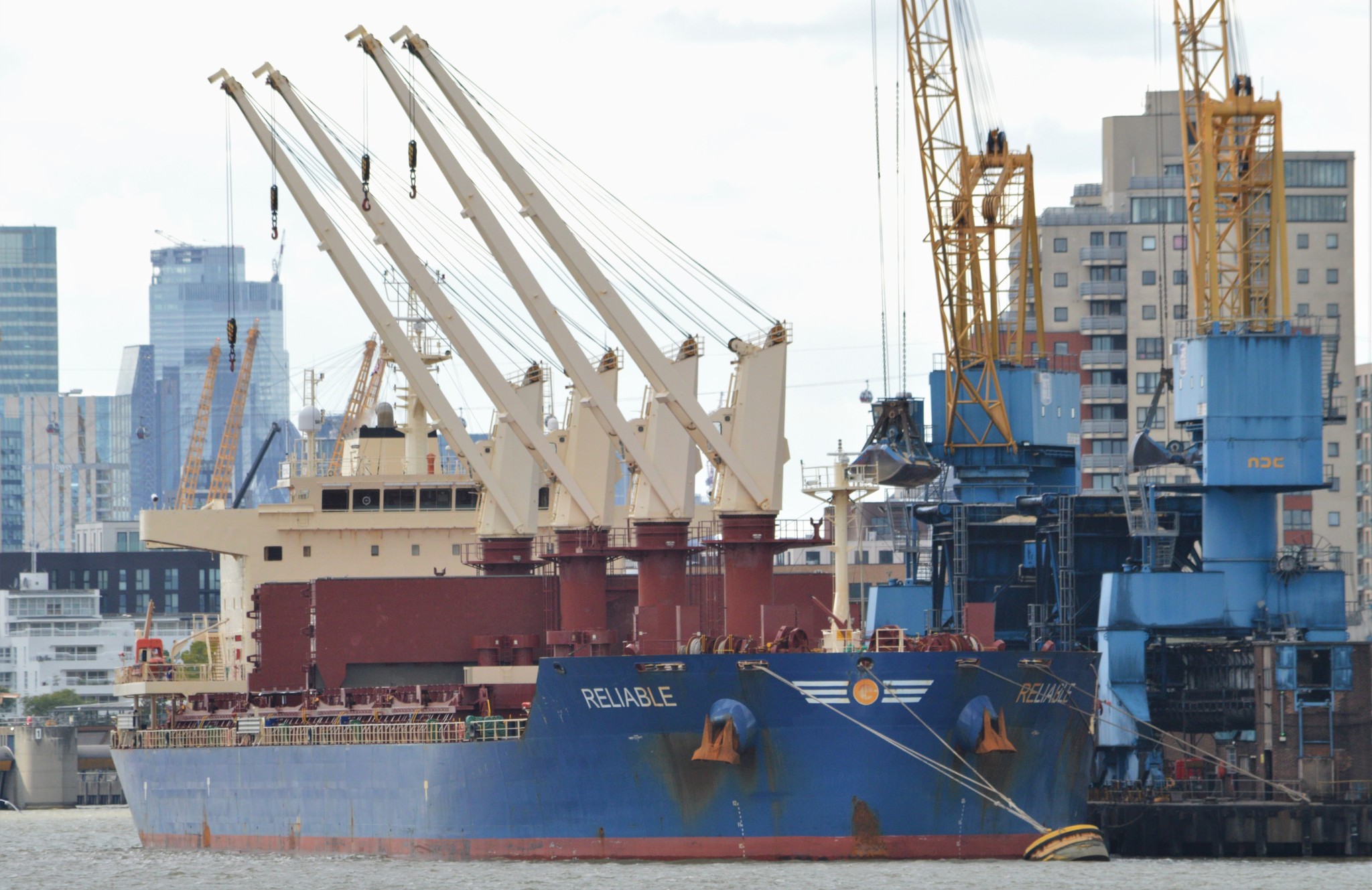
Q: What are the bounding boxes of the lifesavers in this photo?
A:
[1335,752,1351,771]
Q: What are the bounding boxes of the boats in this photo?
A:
[101,19,1119,867]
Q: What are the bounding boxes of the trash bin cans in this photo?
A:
[426,718,441,740]
[278,723,291,743]
[46,720,56,726]
[206,726,220,746]
[349,719,362,744]
